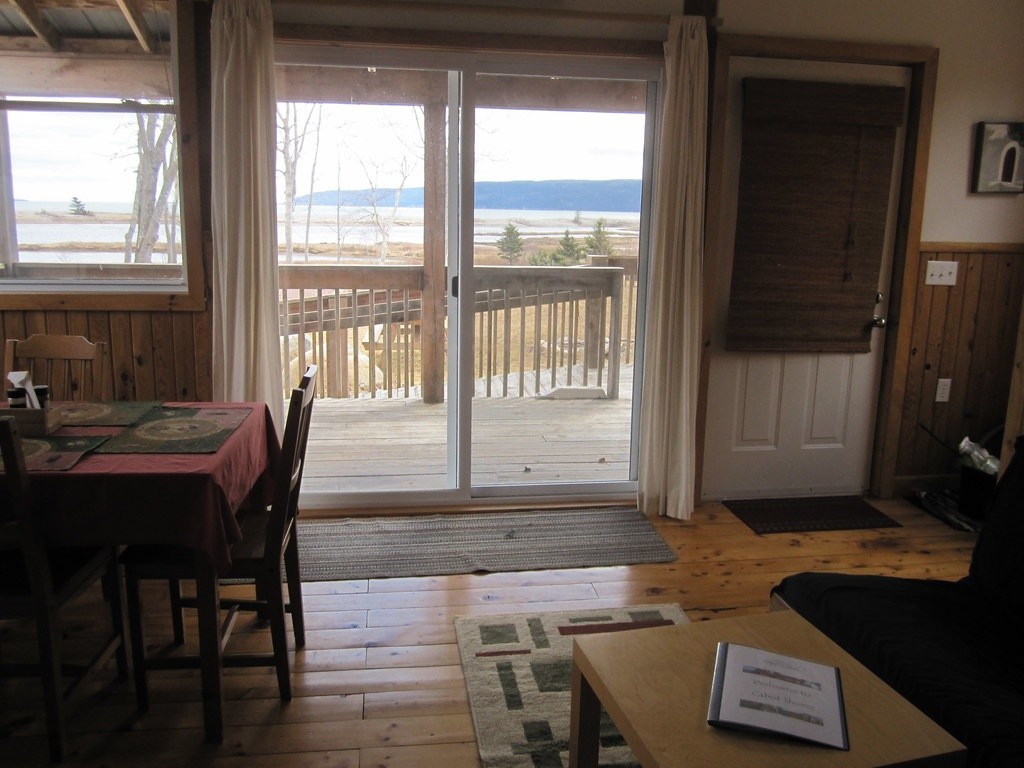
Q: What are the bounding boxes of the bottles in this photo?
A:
[959,436,998,474]
[7,389,27,408]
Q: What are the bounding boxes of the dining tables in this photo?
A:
[0,400,280,743]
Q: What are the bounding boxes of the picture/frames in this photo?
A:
[971,121,1023,193]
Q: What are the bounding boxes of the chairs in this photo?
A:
[0,415,151,768]
[2,335,108,608]
[117,364,318,711]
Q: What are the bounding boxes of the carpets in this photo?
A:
[219,511,677,585]
[452,602,692,768]
[721,495,903,533]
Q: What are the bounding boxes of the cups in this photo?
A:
[34,385,49,411]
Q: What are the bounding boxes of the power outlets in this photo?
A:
[934,379,952,401]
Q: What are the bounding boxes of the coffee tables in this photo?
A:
[569,608,966,767]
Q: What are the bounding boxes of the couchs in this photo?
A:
[770,433,1024,768]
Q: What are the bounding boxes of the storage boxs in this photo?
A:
[0,406,62,435]
